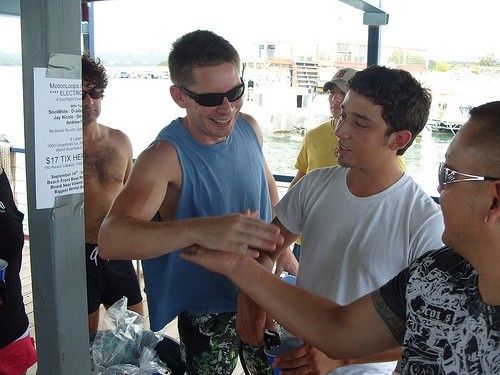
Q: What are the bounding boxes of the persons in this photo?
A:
[288,69,407,276]
[82,55,144,346]
[0,165,37,375]
[180,101,500,375]
[236,65,446,375]
[98,30,299,375]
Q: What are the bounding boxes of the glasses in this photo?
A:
[178,76,244,106]
[438,161,500,186]
[82,85,103,101]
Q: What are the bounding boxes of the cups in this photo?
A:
[282,275,297,286]
[264,337,304,375]
[0,258,8,282]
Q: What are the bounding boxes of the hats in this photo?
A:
[323,68,359,93]
